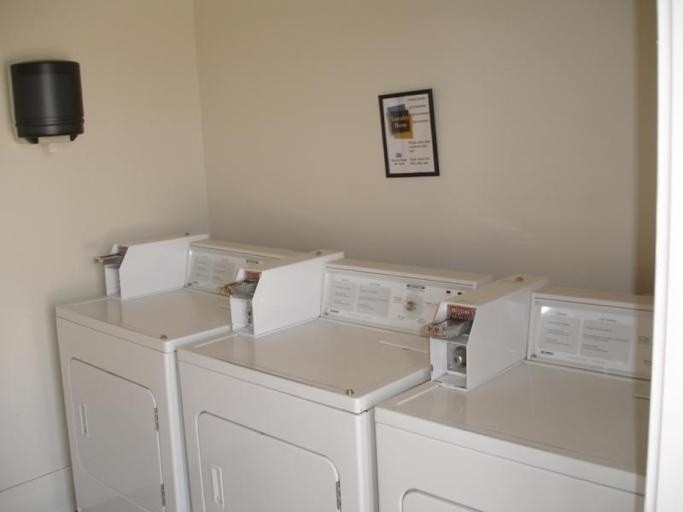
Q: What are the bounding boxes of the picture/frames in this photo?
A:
[378,88,439,178]
